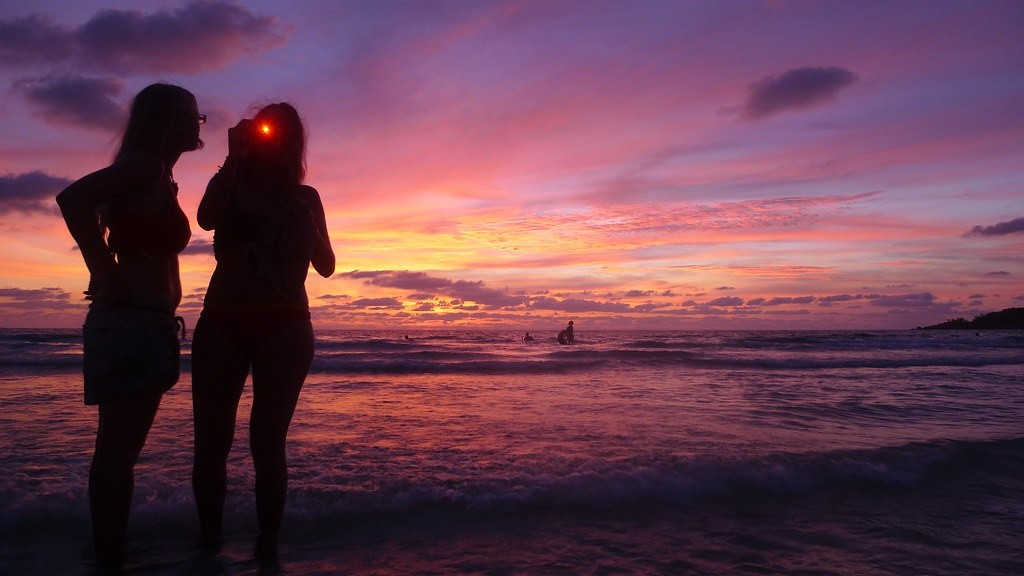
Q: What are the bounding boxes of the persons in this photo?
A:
[524,332,534,341]
[56,82,205,576]
[564,321,574,343]
[190,103,335,576]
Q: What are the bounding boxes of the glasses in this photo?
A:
[171,112,206,125]
